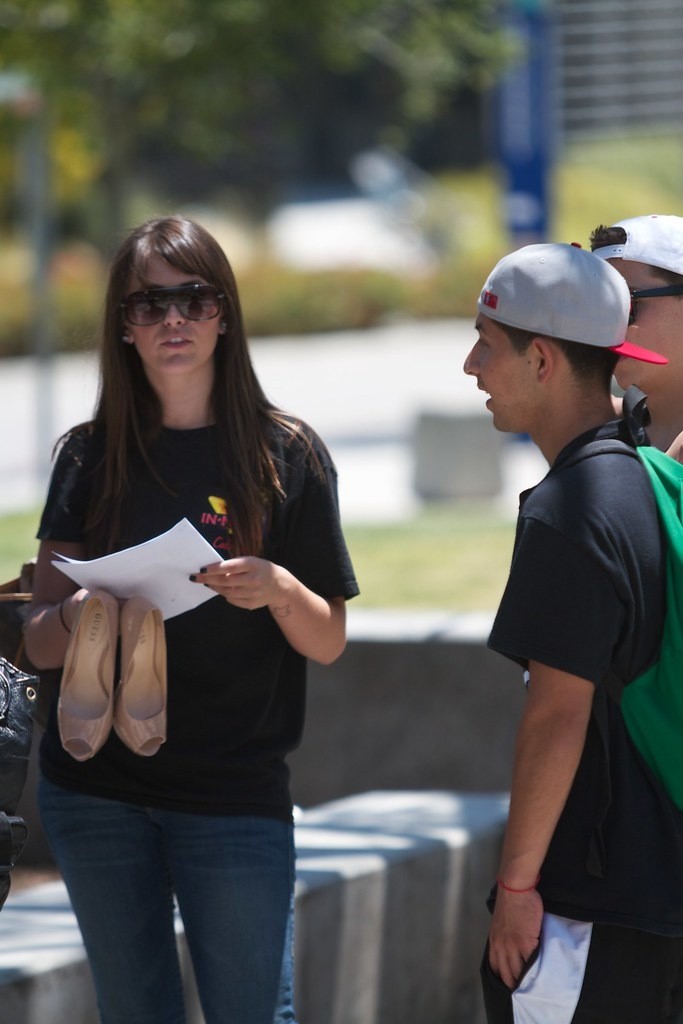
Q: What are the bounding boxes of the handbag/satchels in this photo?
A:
[0,658,40,814]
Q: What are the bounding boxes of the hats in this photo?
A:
[476,243,669,366]
[592,214,683,276]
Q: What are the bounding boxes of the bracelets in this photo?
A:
[497,875,539,894]
[59,597,72,634]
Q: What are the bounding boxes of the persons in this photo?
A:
[463,213,683,1024]
[23,216,360,1024]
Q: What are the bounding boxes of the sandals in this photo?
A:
[113,597,167,756]
[56,589,119,762]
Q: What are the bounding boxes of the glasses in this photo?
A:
[120,284,225,326]
[627,283,682,325]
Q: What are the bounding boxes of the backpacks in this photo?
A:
[614,446,683,808]
